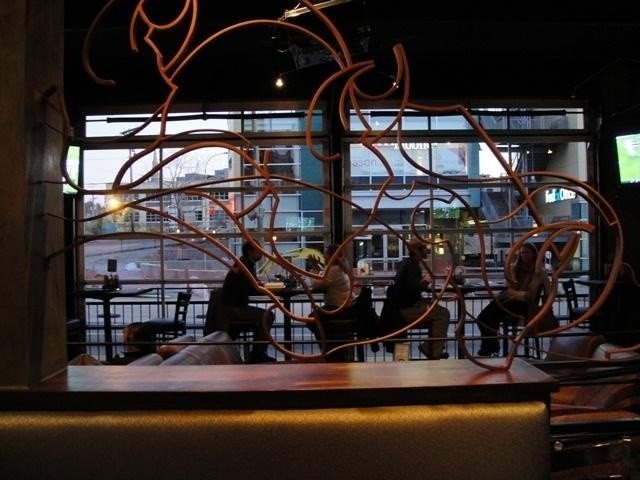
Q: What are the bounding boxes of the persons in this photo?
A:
[222,241,275,361]
[391,235,451,360]
[69,322,157,365]
[475,242,553,358]
[302,243,363,363]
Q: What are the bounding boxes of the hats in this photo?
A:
[408,236,433,245]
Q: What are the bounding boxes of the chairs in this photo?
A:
[201,263,638,364]
[122,288,196,356]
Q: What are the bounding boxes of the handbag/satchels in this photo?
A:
[525,303,560,334]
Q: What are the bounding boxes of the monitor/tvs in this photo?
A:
[61,142,83,197]
[612,129,640,188]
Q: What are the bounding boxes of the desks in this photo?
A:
[70,284,154,362]
